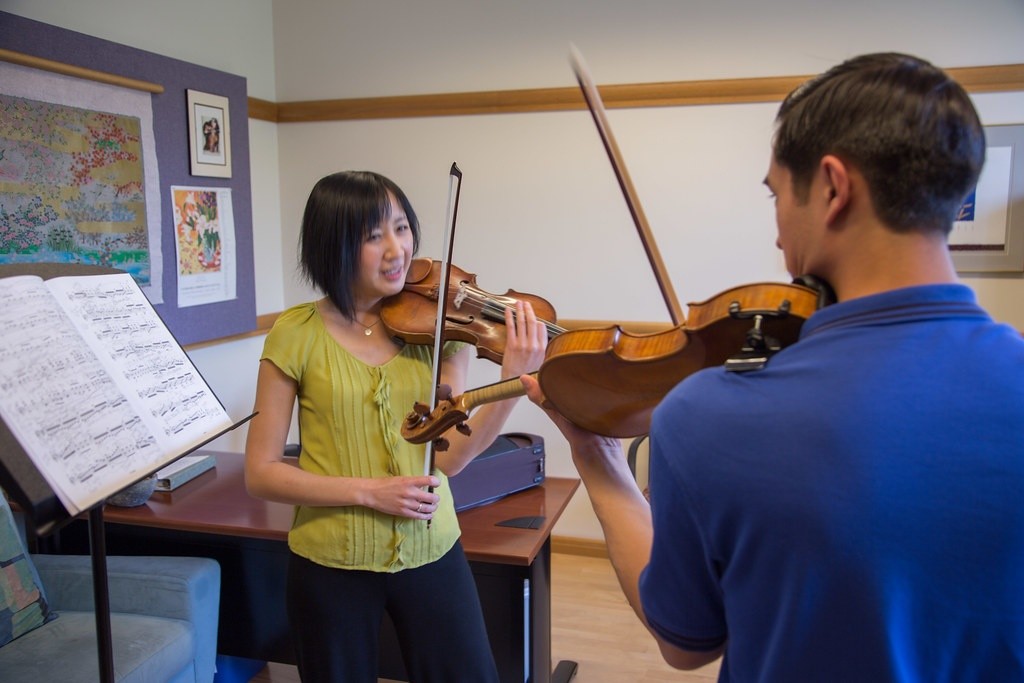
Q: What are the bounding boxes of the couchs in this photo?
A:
[0,502,221,683]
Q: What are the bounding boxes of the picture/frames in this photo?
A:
[185,88,232,178]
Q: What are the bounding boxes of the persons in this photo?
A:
[244,170,549,683]
[520,52,1024,683]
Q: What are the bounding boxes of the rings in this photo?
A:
[416,504,422,511]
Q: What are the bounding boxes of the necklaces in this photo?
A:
[351,316,382,336]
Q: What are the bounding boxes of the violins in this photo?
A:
[380,256,573,368]
[400,277,840,453]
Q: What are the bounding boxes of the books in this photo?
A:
[0,273,236,518]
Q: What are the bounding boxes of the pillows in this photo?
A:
[0,488,60,648]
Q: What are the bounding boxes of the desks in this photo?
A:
[77,448,581,683]
